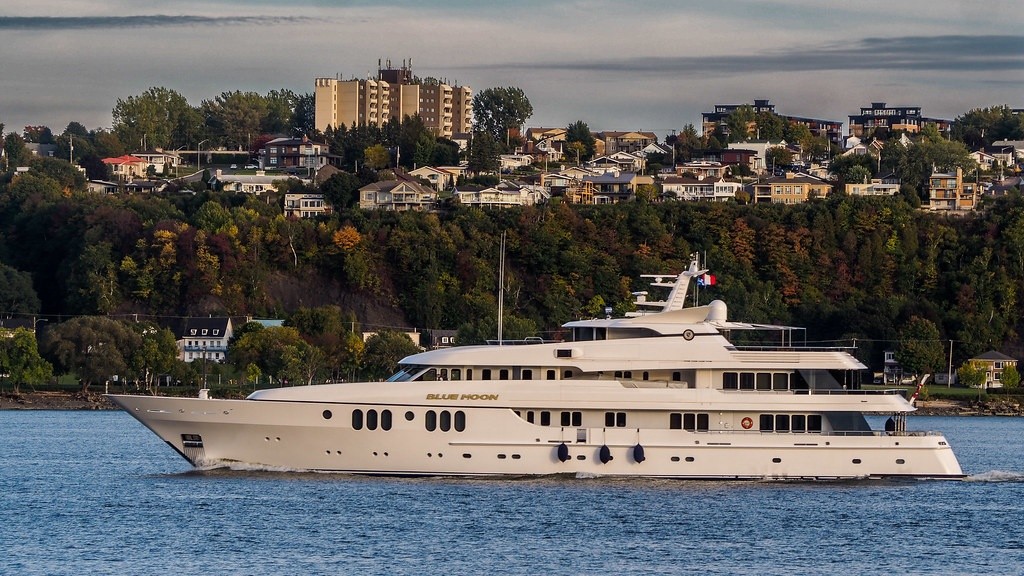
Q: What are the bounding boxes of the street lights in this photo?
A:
[202,346,206,389]
[34,317,48,337]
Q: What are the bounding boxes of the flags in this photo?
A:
[697,274,717,286]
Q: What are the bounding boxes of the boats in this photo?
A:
[99,252,969,482]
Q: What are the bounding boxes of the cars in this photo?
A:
[325,377,345,383]
[901,378,913,385]
[872,378,883,384]
[153,374,179,386]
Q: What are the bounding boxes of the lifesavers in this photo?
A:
[742,417,753,429]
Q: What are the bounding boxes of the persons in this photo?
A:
[437,374,443,381]
[443,373,448,381]
